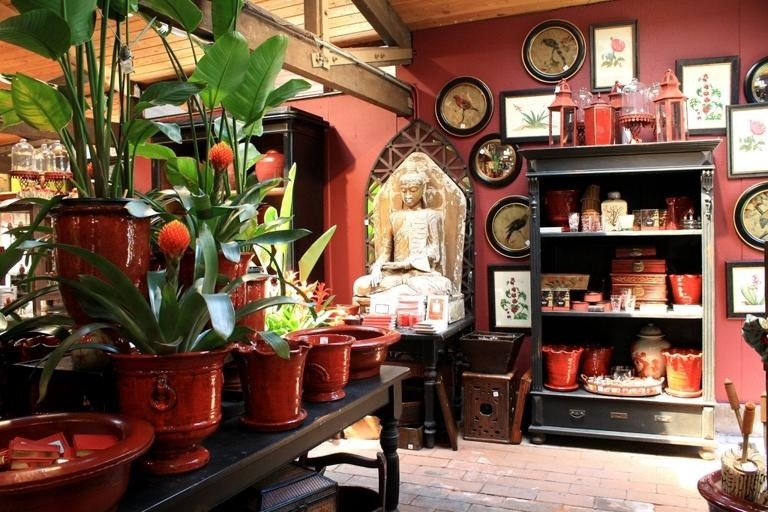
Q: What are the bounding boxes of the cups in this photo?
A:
[610,287,637,313]
[568,212,634,232]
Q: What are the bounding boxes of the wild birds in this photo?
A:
[453,94,479,112]
[504,214,528,244]
[541,38,566,66]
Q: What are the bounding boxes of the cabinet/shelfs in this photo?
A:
[517,139,723,460]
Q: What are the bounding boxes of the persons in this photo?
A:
[353,171,452,296]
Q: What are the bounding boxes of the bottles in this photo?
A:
[611,366,632,382]
[10,138,72,171]
[573,77,662,144]
[601,192,628,231]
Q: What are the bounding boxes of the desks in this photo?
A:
[398,316,476,448]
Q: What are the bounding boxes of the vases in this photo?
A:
[541,343,584,392]
[660,348,703,398]
[580,345,615,376]
